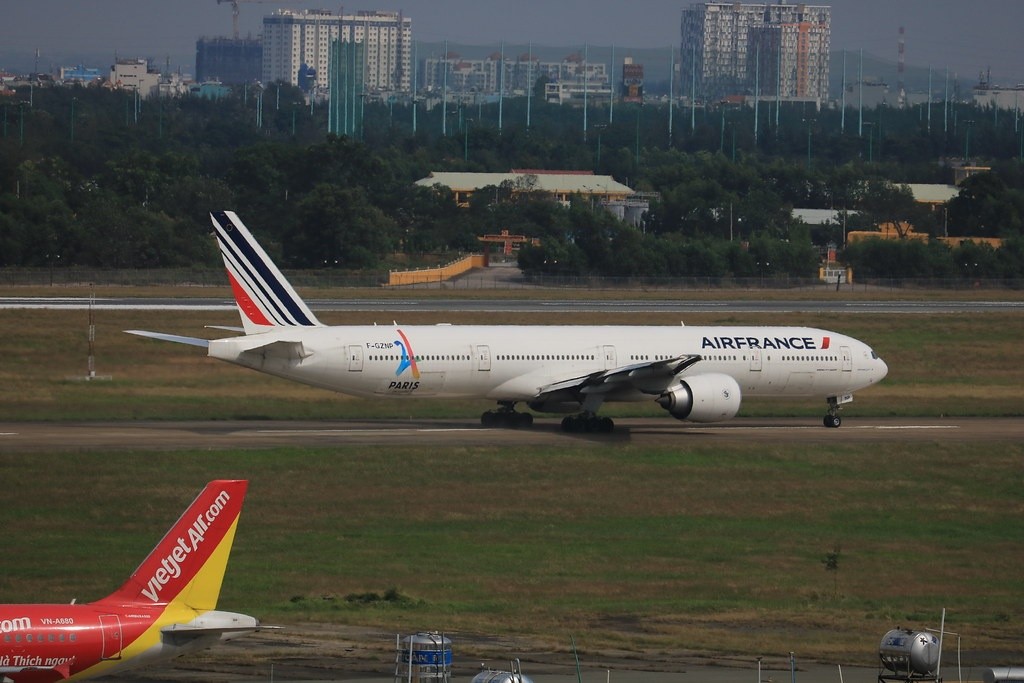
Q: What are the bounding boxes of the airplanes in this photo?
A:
[124,209,891,434]
[0,480,287,683]
